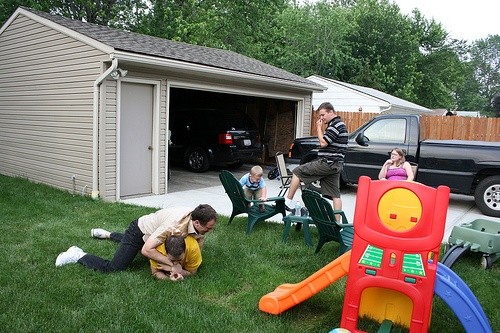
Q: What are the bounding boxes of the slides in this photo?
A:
[259,248,352,315]
[434,261,494,333]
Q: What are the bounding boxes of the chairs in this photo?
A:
[302,190,354,256]
[219,170,286,235]
[275,151,306,197]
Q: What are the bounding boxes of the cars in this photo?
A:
[166,103,264,173]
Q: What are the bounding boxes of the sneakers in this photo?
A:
[55,244,84,267]
[90,227,103,238]
[273,198,292,210]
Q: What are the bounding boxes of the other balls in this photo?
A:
[329,328,352,333]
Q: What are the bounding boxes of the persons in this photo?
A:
[54,204,219,273]
[148,235,203,281]
[238,166,267,213]
[378,147,415,180]
[446,107,457,115]
[282,102,349,232]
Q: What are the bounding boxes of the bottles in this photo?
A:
[295,201,301,216]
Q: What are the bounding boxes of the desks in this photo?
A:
[282,207,315,245]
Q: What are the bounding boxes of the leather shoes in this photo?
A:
[259,205,266,213]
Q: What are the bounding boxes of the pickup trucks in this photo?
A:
[288,112,496,218]
